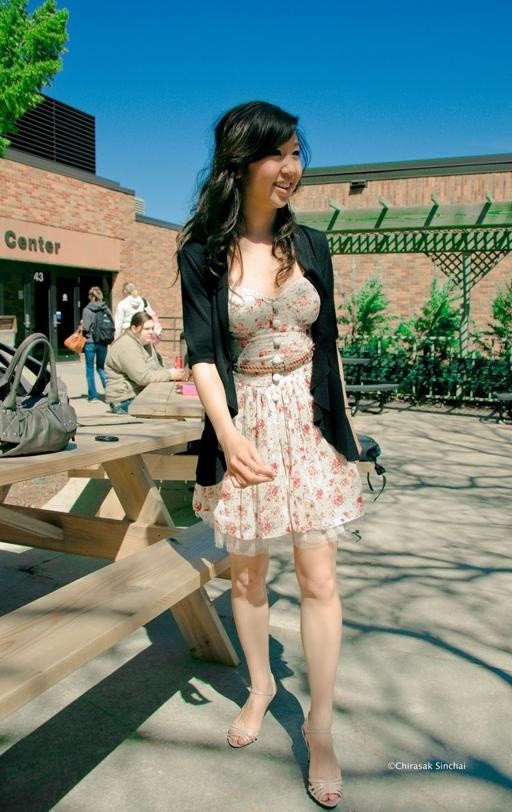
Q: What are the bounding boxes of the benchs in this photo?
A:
[0,521,234,722]
[484,391,512,423]
[345,383,400,415]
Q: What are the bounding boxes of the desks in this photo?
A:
[341,357,371,406]
[0,411,242,668]
[128,379,205,454]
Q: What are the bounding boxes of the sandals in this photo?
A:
[300,712,343,808]
[227,675,277,749]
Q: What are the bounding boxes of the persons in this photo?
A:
[112,281,164,345]
[103,311,193,457]
[168,98,367,812]
[76,284,115,403]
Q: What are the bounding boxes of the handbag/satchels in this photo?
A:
[0,397,80,458]
[64,331,87,353]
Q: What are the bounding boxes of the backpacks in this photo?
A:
[89,308,115,346]
